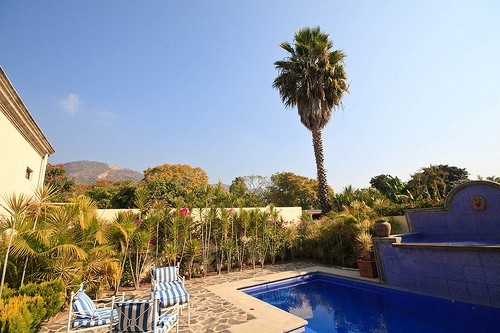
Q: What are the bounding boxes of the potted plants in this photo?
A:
[373,198,393,237]
[355,235,379,279]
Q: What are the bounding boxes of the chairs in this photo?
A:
[68,262,191,333]
